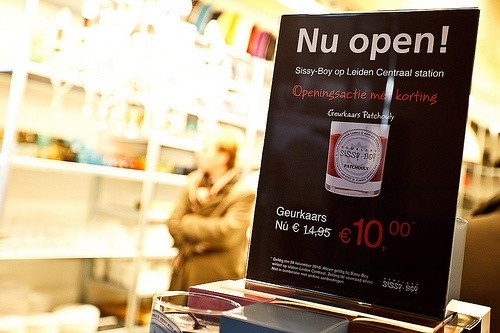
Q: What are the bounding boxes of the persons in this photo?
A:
[33,140,80,164]
[159,130,256,310]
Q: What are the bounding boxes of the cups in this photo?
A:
[148,291,241,333]
